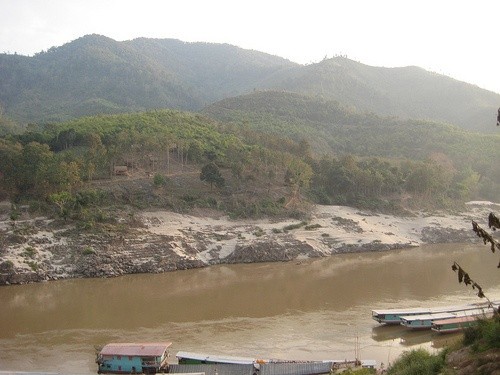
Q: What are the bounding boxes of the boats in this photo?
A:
[432,312,500,334]
[92,341,378,375]
[399,306,499,330]
[372,300,500,326]
